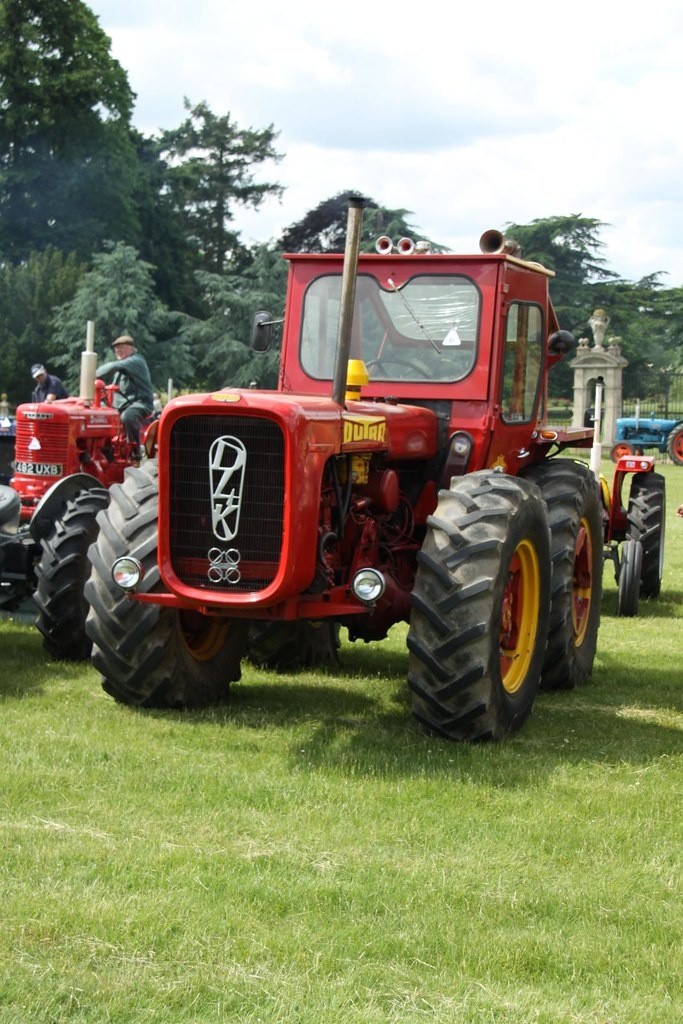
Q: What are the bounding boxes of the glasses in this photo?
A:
[36,373,44,379]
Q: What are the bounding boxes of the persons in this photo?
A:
[95,335,154,461]
[30,365,70,405]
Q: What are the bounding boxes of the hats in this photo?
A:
[30,363,45,379]
[110,336,135,346]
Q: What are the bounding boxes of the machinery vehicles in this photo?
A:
[0,319,161,663]
[84,209,604,745]
[610,410,683,466]
